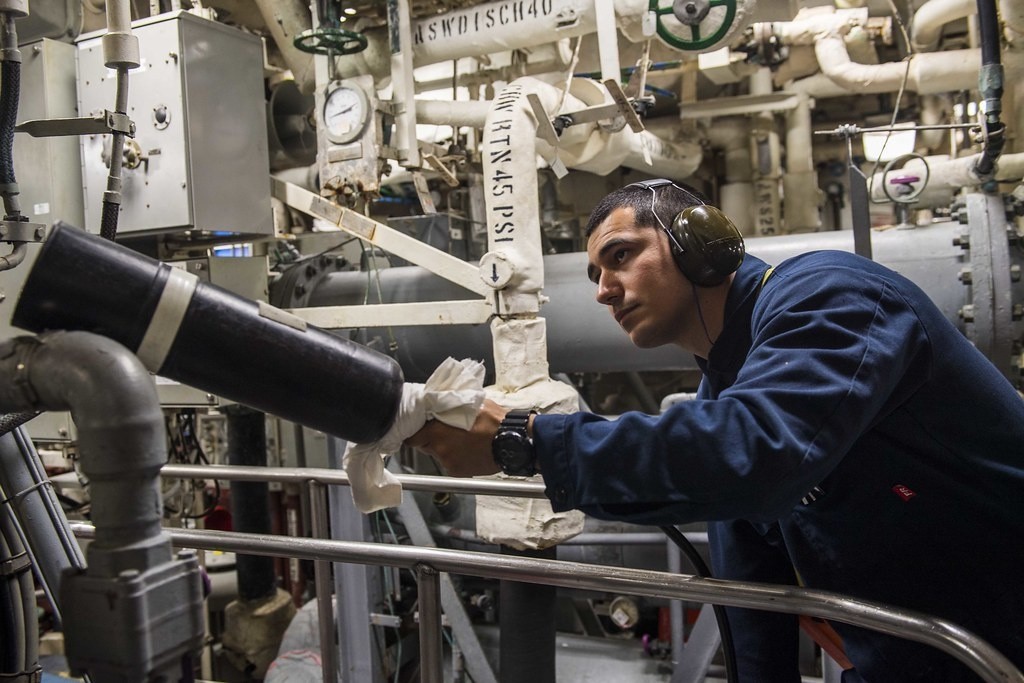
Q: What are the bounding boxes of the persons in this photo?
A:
[404,178,1024,683]
[491,408,538,478]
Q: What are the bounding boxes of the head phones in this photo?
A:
[624,178,745,287]
